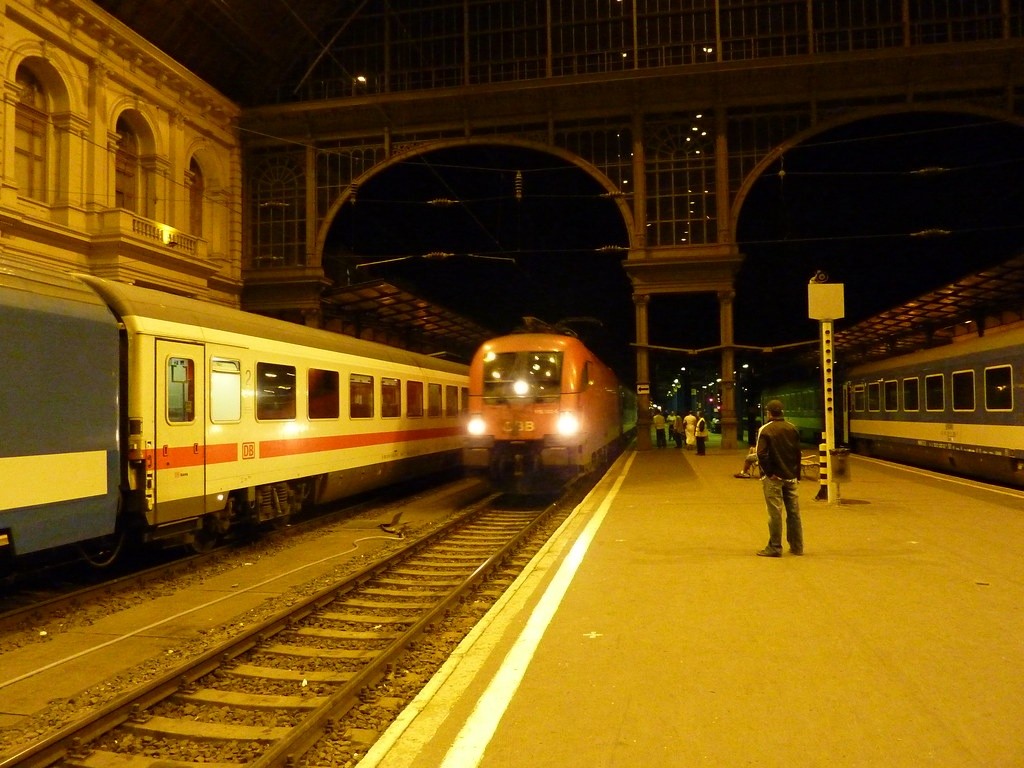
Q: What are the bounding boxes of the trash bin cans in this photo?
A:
[829,448,851,484]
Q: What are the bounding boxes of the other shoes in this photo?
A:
[789,548,803,555]
[757,550,781,557]
[733,473,751,478]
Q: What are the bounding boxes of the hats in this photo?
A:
[765,400,783,411]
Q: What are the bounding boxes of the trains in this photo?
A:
[843,321,1024,485]
[0,254,468,586]
[468,333,623,484]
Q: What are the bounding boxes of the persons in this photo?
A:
[668,410,677,443]
[654,409,668,448]
[673,410,685,450]
[696,410,710,456]
[683,410,698,450]
[735,452,761,479]
[756,399,806,557]
